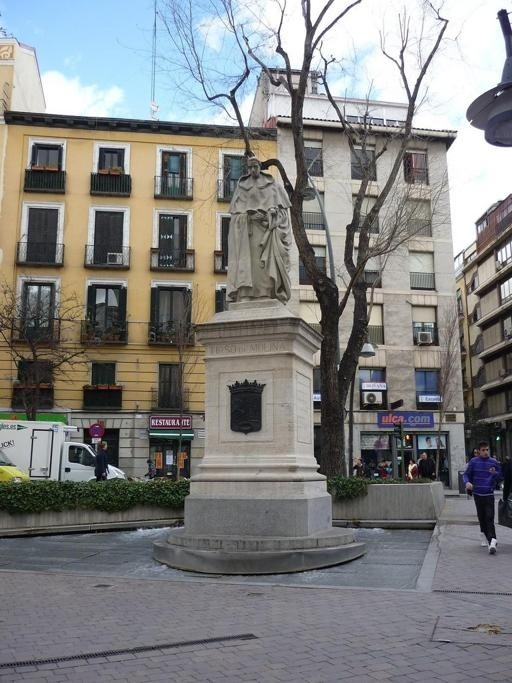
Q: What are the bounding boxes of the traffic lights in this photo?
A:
[405,433,412,448]
[393,420,402,439]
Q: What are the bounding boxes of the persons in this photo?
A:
[351,457,368,476]
[418,452,435,480]
[94,440,110,481]
[376,461,390,481]
[465,446,511,501]
[463,441,505,554]
[407,458,418,479]
[423,436,434,448]
[75,447,83,463]
[228,154,291,300]
[144,459,157,478]
[378,434,389,448]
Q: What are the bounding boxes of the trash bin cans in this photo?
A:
[458,471,467,494]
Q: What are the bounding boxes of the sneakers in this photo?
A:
[480,532,489,547]
[487,537,497,554]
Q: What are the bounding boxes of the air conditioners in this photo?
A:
[417,331,432,346]
[362,391,383,407]
[107,252,124,265]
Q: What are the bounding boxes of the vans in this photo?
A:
[1,449,32,484]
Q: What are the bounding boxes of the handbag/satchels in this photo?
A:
[498,498,512,528]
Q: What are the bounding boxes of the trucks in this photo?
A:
[0,418,128,481]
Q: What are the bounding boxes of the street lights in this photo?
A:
[347,334,377,477]
[466,9,511,147]
